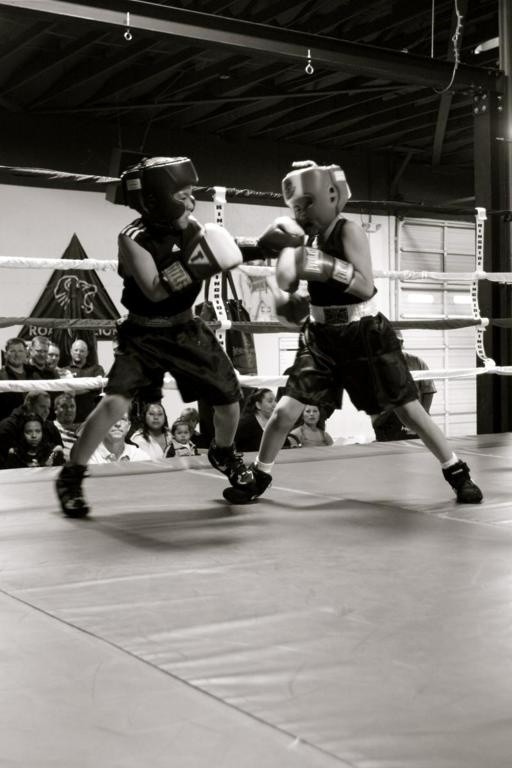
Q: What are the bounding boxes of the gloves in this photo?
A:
[277,245,354,293]
[163,223,243,293]
[234,217,305,261]
[275,287,310,324]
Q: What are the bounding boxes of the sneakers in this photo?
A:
[208,439,256,487]
[56,463,88,516]
[442,459,482,503]
[223,461,272,500]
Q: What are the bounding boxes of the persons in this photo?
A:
[222,159,483,501]
[53,155,305,519]
[369,329,435,443]
[231,390,334,454]
[1,328,206,470]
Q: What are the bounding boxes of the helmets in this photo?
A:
[120,157,198,224]
[282,163,351,233]
